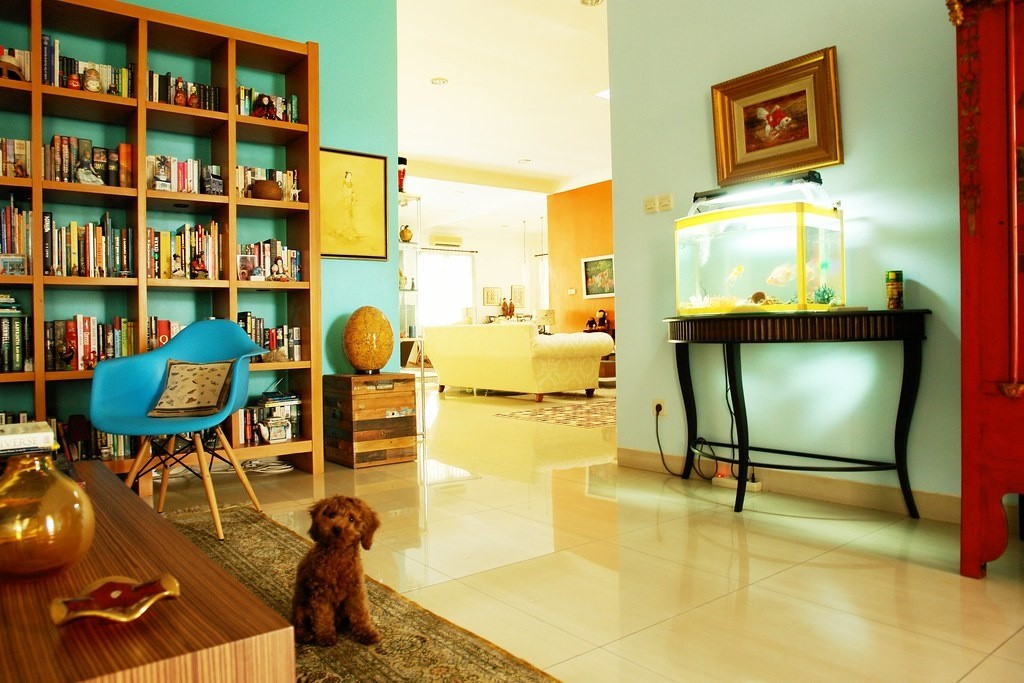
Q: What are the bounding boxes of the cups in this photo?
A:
[409,326,416,338]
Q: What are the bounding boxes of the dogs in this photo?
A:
[290,494,383,649]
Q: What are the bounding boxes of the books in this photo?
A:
[0,36,303,128]
[0,295,307,464]
[1,195,309,284]
[1,136,307,208]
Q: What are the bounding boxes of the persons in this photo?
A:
[508,299,514,317]
[502,298,508,316]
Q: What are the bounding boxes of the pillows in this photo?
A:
[145,358,237,417]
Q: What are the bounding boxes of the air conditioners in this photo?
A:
[428,236,463,248]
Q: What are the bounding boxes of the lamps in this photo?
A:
[534,308,555,336]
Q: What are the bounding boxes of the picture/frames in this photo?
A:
[0,254,27,276]
[710,44,845,189]
[580,255,614,299]
[319,145,388,261]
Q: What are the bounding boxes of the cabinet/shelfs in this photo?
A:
[0,1,325,478]
[398,191,427,440]
[324,373,417,469]
[951,0,1024,578]
[582,327,616,387]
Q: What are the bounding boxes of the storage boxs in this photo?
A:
[673,166,846,316]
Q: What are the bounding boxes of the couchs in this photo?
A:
[423,320,616,404]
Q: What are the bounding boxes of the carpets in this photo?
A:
[152,500,564,682]
[489,394,616,431]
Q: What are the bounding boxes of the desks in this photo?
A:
[0,457,295,683]
[661,308,933,521]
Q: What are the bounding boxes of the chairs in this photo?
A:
[90,318,272,541]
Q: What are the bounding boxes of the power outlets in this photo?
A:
[652,399,666,417]
